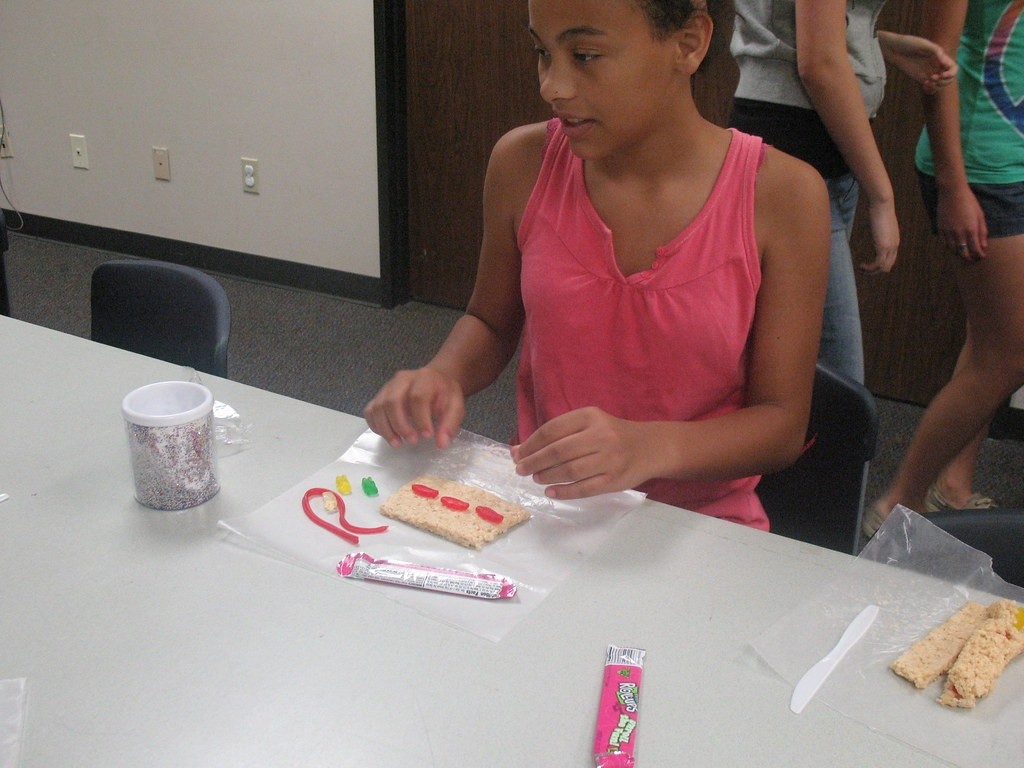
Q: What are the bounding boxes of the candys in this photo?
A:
[412,484,503,523]
[323,474,378,513]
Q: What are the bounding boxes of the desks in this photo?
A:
[0,313,1024,768]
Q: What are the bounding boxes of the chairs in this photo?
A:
[91,259,232,378]
[757,357,880,559]
[868,508,1024,604]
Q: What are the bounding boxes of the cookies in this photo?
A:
[380,473,532,549]
[889,599,1024,709]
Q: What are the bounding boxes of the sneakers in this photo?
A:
[924,482,996,511]
[862,502,886,538]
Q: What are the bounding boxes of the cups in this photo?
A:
[121,381,221,509]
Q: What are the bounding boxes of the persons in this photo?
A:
[862,0,1024,540]
[727,0,959,385]
[364,0,832,532]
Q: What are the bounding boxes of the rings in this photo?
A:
[959,243,967,249]
